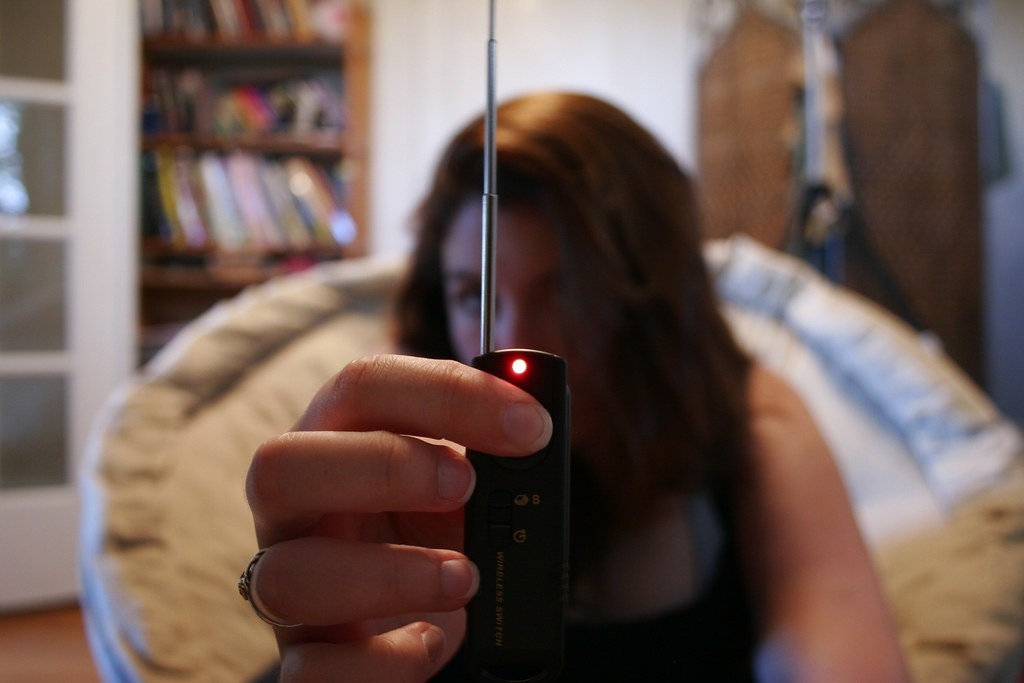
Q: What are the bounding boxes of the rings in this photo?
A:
[237,548,304,628]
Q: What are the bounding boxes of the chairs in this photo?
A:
[82,234,1024,683]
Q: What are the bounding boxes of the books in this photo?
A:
[139,0,352,247]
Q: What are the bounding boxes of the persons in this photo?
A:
[237,90,914,683]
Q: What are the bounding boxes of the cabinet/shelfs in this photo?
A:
[140,0,372,367]
[0,0,140,612]
